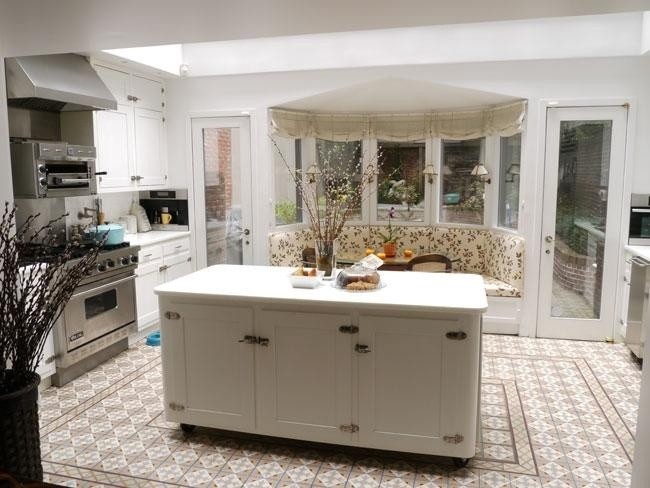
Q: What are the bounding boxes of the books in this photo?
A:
[349,254,384,269]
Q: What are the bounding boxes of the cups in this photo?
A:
[365,247,412,259]
[153,216,160,224]
[161,214,172,224]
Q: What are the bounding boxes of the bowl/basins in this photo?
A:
[145,330,161,346]
[288,267,326,288]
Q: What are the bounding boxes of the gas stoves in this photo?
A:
[14,241,140,282]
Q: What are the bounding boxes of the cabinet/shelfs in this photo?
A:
[618,245,633,338]
[122,228,196,331]
[152,262,488,466]
[57,55,168,191]
[0,256,58,395]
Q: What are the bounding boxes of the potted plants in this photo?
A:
[376,220,400,258]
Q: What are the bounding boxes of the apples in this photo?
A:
[366,249,375,256]
[377,252,386,260]
[404,250,412,257]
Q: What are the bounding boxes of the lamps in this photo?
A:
[421,163,439,184]
[442,164,454,182]
[504,163,522,184]
[363,163,379,183]
[469,163,492,185]
[306,162,321,184]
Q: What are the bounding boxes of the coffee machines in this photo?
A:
[138,191,189,232]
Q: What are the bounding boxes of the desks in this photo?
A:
[333,251,462,271]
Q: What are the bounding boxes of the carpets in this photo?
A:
[37,311,648,488]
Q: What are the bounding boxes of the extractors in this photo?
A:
[4,53,118,116]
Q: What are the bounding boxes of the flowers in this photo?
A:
[263,129,407,266]
[0,200,113,397]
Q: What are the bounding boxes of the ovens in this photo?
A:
[628,192,650,247]
[48,270,140,368]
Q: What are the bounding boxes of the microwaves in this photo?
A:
[9,136,98,198]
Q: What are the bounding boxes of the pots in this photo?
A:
[443,192,460,206]
[88,221,125,245]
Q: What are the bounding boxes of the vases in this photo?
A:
[1,368,42,488]
[314,238,341,278]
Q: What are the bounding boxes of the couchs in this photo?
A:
[265,220,525,334]
